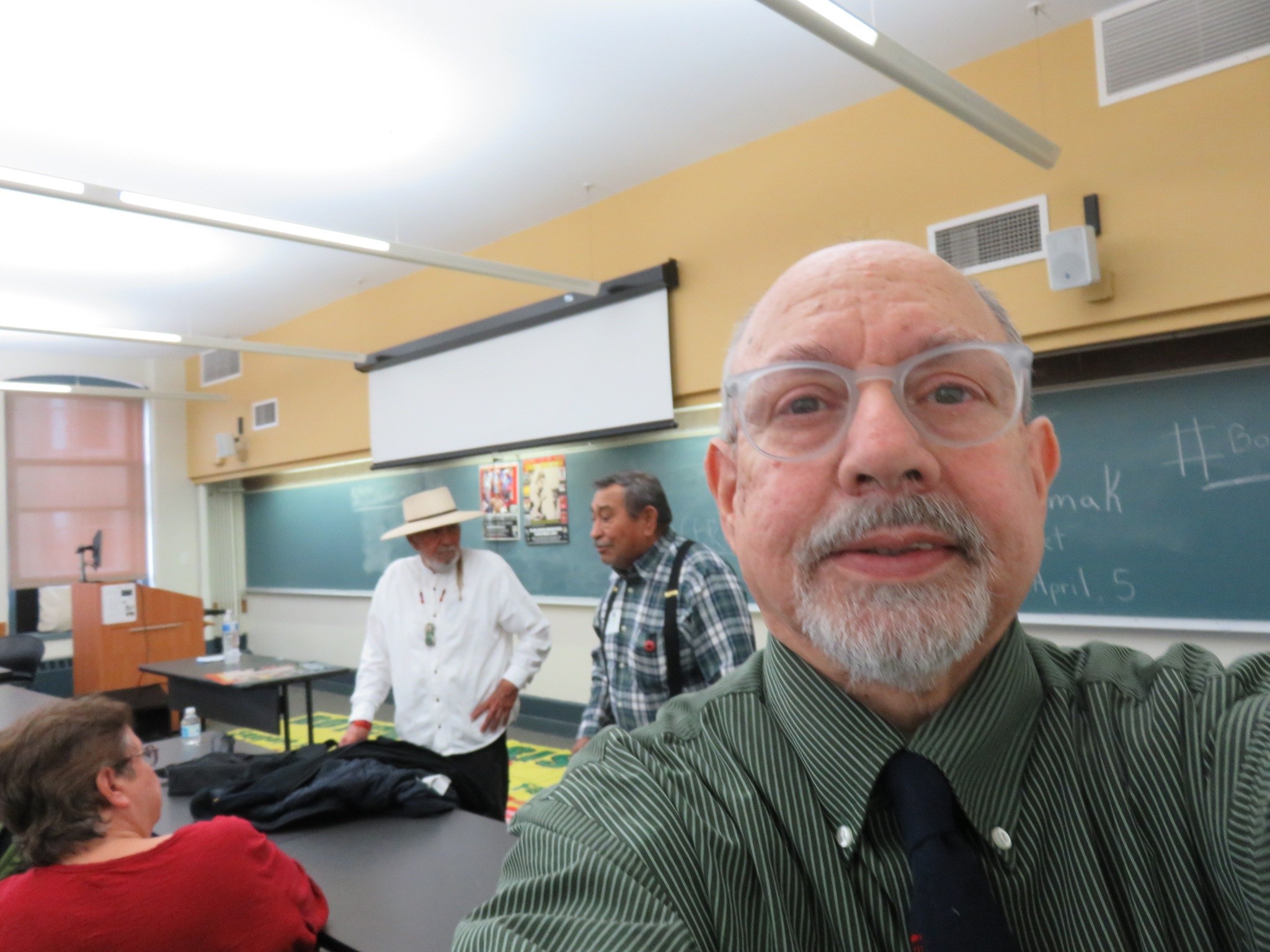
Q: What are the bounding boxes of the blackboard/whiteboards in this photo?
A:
[242,356,1270,633]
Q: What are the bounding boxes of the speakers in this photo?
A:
[214,431,236,459]
[1044,225,1100,292]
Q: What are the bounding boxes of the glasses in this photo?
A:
[93,742,159,791]
[725,341,1031,462]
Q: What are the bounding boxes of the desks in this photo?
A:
[0,683,70,731]
[143,729,519,952]
[139,651,351,750]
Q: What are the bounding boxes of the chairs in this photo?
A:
[0,635,45,689]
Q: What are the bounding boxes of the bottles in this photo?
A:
[222,610,239,664]
[181,707,202,762]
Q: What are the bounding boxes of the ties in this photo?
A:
[879,749,1014,952]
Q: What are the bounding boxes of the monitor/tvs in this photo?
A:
[91,528,102,568]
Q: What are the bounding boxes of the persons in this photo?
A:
[451,238,1270,952]
[568,470,756,755]
[339,486,552,823]
[0,692,330,952]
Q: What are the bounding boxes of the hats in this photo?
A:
[380,486,487,541]
